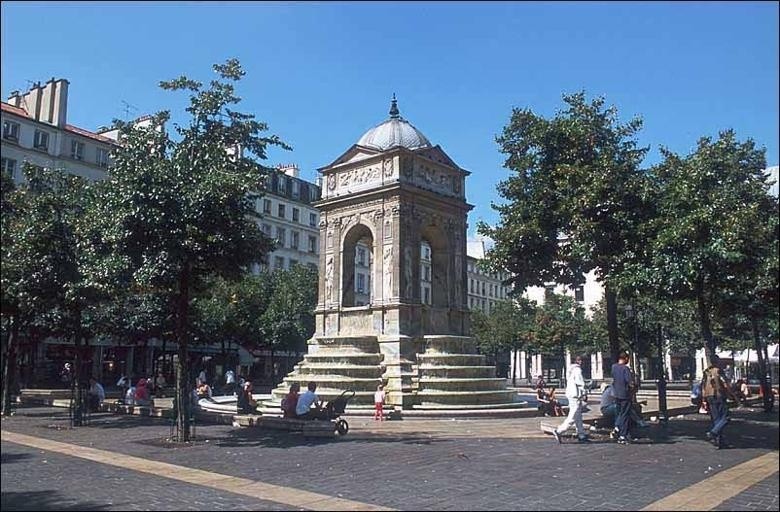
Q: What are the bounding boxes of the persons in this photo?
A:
[199,381,213,399]
[548,386,567,415]
[135,378,154,410]
[296,381,327,421]
[527,372,533,383]
[198,367,208,385]
[192,384,200,405]
[728,379,749,408]
[535,375,546,389]
[629,385,648,425]
[236,374,246,389]
[59,362,73,389]
[690,381,709,414]
[225,367,236,395]
[663,367,670,381]
[85,377,106,409]
[373,384,386,421]
[237,381,262,415]
[124,385,137,405]
[552,355,592,442]
[609,351,635,444]
[155,371,166,397]
[280,383,301,419]
[699,354,741,449]
[739,380,751,396]
[535,386,552,415]
[599,380,620,429]
[722,363,733,382]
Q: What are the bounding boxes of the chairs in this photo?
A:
[704,430,737,450]
[552,429,629,445]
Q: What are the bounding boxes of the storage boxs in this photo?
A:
[92,399,337,437]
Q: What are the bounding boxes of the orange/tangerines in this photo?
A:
[312,390,355,434]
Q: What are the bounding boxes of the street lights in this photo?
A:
[698,400,710,416]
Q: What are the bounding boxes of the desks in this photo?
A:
[625,304,641,413]
